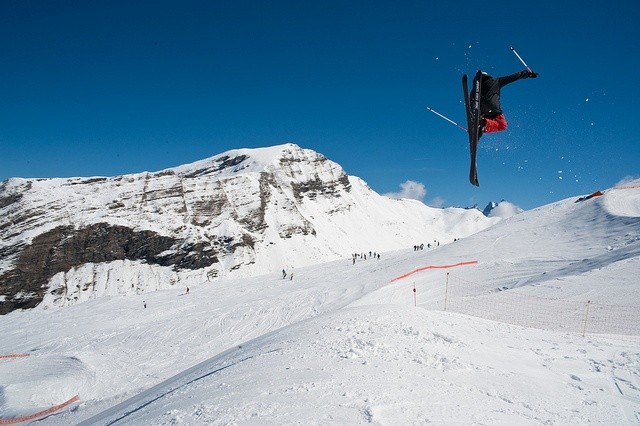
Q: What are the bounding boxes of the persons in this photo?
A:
[468,70,539,141]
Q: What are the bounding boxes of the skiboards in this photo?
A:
[462,70,482,187]
